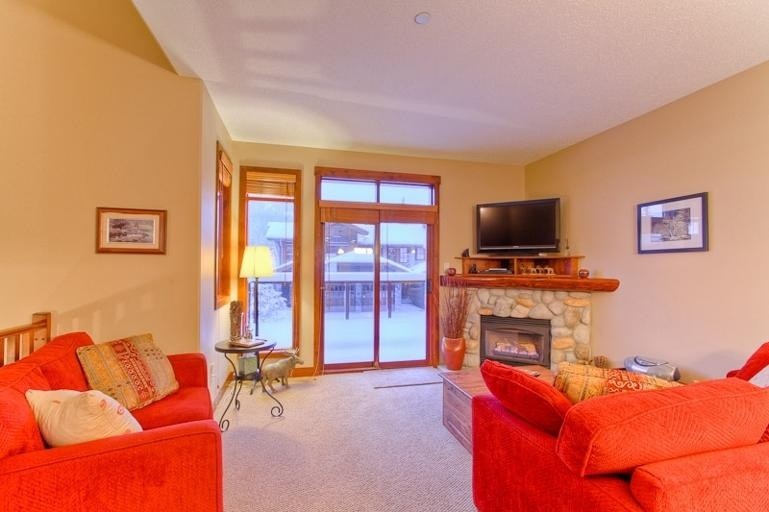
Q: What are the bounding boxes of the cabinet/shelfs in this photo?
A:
[454,251,585,276]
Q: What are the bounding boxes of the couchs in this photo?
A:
[468,343,768,512]
[0,325,223,512]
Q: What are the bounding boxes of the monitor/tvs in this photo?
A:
[476,197,561,256]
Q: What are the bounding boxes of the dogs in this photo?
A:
[249,353,304,395]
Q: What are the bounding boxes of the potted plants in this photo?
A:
[437,270,475,370]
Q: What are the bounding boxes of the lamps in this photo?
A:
[238,243,280,394]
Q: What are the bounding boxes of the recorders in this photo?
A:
[622,355,681,383]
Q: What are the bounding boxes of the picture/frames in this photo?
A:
[95,204,168,255]
[636,191,709,255]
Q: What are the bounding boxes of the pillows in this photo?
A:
[548,362,689,405]
[24,389,144,446]
[76,332,181,411]
